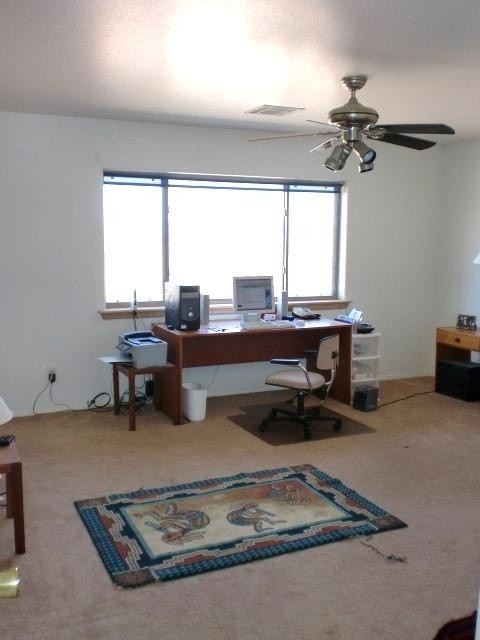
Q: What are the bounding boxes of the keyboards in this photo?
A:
[240,321,295,330]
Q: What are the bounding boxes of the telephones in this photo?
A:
[292,306,321,321]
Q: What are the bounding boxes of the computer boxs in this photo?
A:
[164,281,201,331]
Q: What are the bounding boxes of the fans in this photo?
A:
[246,76,455,173]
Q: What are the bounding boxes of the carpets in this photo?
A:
[74,463,405,589]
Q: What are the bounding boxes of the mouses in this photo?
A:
[297,320,305,326]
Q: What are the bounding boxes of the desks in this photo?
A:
[435,328,480,403]
[153,319,353,426]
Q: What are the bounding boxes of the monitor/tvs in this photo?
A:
[232,276,274,321]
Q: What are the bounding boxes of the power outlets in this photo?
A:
[47,368,58,384]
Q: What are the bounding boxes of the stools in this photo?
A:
[113,364,182,431]
[0,439,26,554]
[437,359,480,403]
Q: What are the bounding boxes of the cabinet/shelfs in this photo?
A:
[354,332,381,405]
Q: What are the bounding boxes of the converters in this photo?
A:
[49,374,56,383]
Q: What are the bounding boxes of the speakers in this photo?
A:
[202,295,209,324]
[277,291,288,320]
[354,385,379,411]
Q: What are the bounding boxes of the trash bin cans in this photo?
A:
[182,382,207,422]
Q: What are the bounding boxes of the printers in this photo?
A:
[99,331,167,369]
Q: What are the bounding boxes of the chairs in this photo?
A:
[260,334,343,439]
